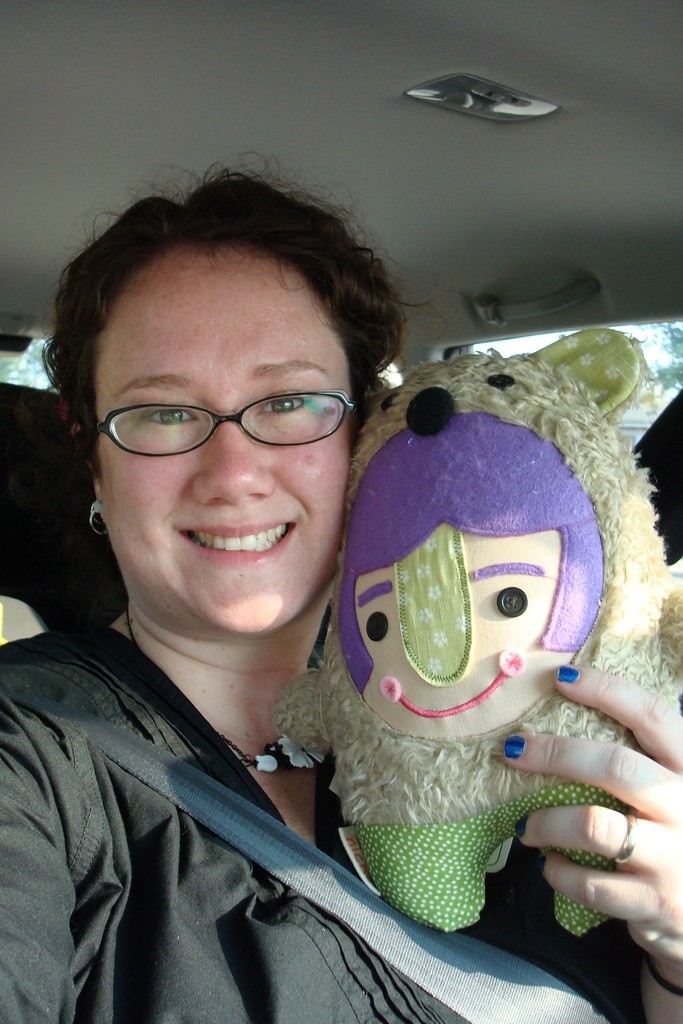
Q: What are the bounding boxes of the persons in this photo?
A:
[0,167,683,1024]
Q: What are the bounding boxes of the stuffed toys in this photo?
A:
[270,326,682,935]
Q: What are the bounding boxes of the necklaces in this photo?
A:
[126,600,328,772]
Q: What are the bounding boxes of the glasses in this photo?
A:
[96,390,357,457]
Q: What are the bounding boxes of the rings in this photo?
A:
[615,815,640,862]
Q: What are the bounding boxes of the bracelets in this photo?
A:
[644,952,683,996]
